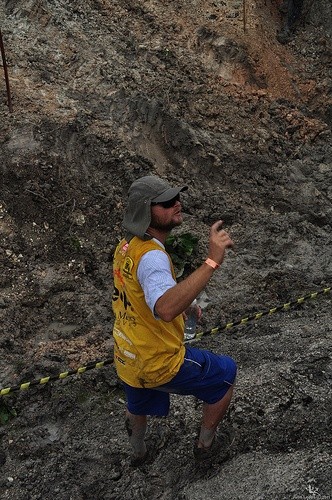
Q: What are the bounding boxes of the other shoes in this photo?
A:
[130,424,172,466]
[194,433,229,462]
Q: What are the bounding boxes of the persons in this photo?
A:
[110,176,237,469]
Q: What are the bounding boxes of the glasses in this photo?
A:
[152,192,180,208]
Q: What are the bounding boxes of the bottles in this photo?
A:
[183,298,197,339]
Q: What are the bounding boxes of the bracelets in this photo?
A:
[204,257,219,271]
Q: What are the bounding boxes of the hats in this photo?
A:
[121,176,188,238]
